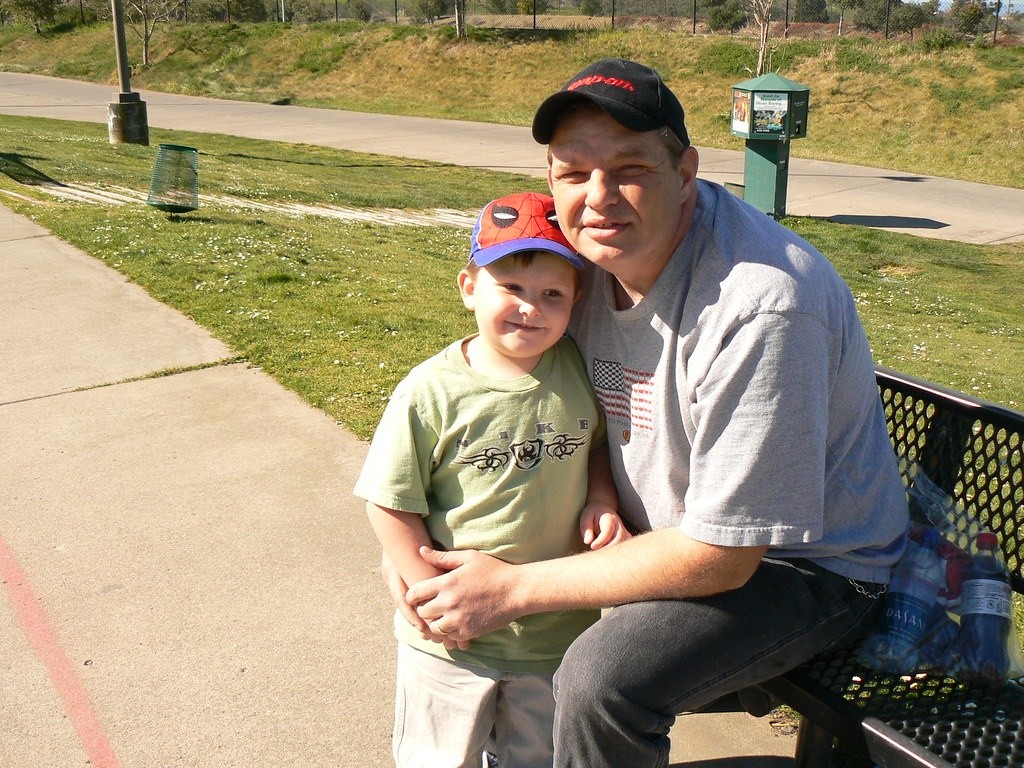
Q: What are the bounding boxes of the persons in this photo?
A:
[353,193,630,768]
[382,61,909,768]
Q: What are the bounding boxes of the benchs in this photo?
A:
[688,366,1024,768]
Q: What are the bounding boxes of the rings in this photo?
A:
[436,620,448,634]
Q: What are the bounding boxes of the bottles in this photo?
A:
[960,533,1012,680]
[858,528,942,674]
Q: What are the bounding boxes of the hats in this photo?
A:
[470,192,587,271]
[532,58,690,150]
